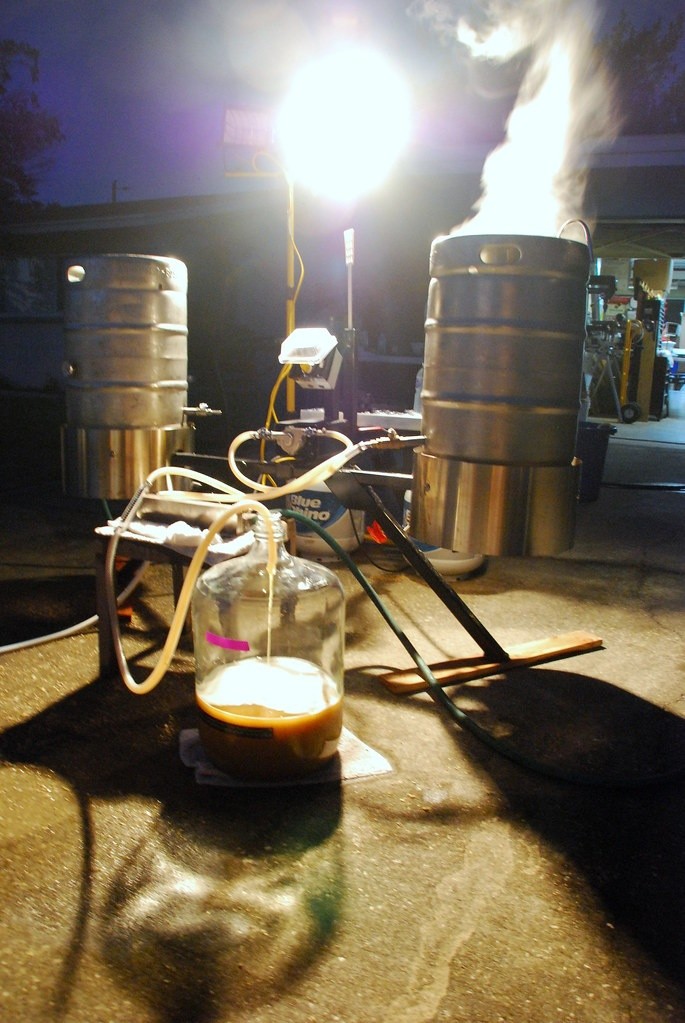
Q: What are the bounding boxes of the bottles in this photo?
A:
[189,510,346,768]
